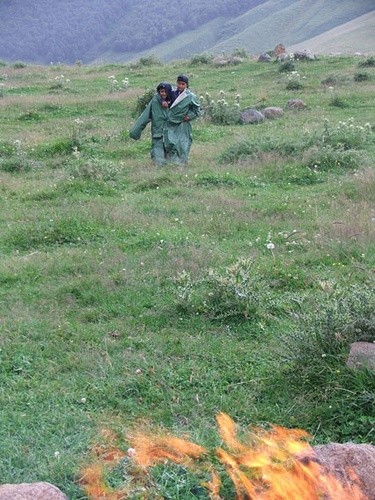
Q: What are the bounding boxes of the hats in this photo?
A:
[157,82,172,91]
[177,75,188,83]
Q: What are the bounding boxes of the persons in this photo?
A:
[156,74,200,164]
[129,83,173,163]
